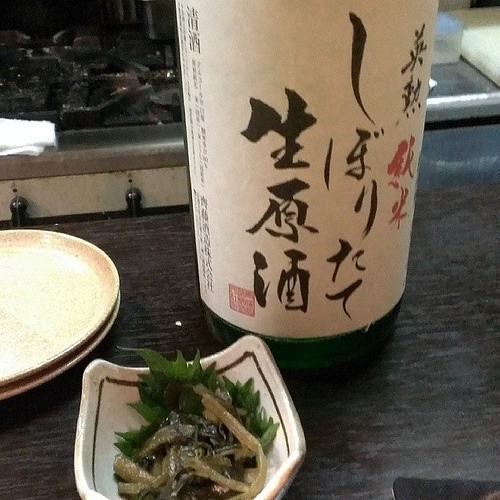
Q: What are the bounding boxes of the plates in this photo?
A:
[0,229,122,404]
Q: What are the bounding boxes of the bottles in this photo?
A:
[175,0,440,339]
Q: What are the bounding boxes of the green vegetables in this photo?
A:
[110,348,282,470]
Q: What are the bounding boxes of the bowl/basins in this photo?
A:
[69,333,308,500]
